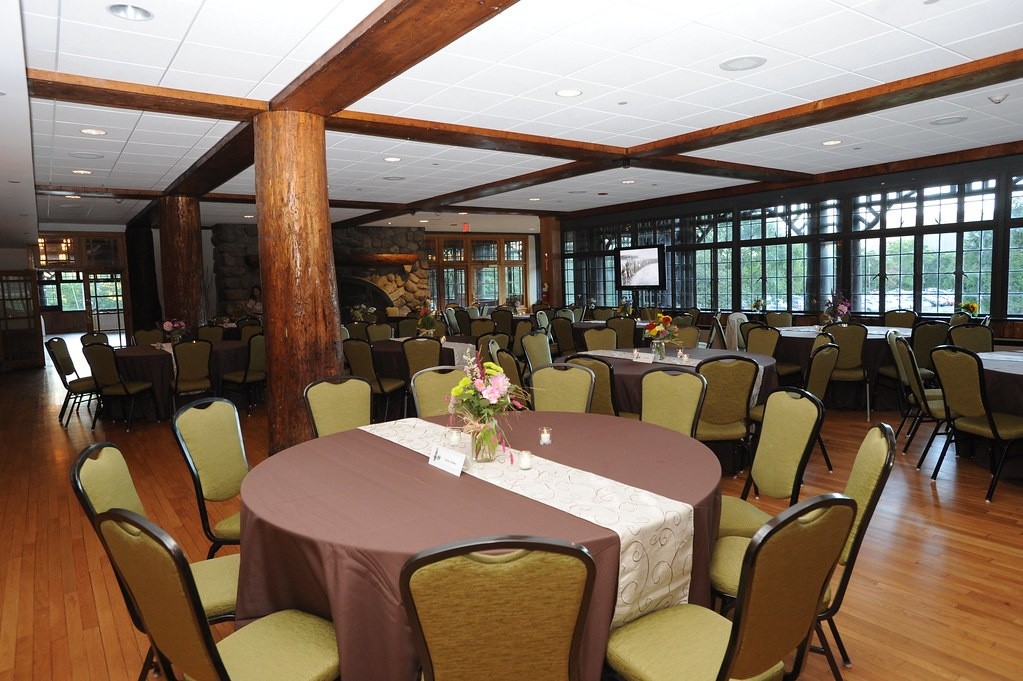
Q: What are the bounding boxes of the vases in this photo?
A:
[470,416,499,466]
[170,334,181,348]
[651,340,666,360]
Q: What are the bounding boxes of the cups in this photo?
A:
[682,354,689,362]
[448,430,461,447]
[677,348,684,358]
[814,325,819,330]
[518,449,532,469]
[632,349,639,357]
[538,427,552,445]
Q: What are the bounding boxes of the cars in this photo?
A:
[822,285,956,316]
[766,294,804,312]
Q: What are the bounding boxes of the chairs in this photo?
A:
[38,302,1023,681]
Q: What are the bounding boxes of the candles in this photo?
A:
[842,324,848,327]
[676,349,684,358]
[633,349,640,358]
[683,353,689,363]
[519,450,532,471]
[538,427,552,444]
[814,325,820,330]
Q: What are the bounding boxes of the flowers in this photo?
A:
[642,313,679,343]
[163,321,191,336]
[445,346,526,427]
[825,289,850,319]
[416,307,437,334]
[959,302,979,317]
[615,298,632,316]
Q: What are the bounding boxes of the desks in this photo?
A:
[106,340,254,421]
[966,350,1023,421]
[569,318,649,350]
[365,335,481,376]
[552,347,776,425]
[773,324,914,398]
[467,314,533,337]
[237,409,723,681]
